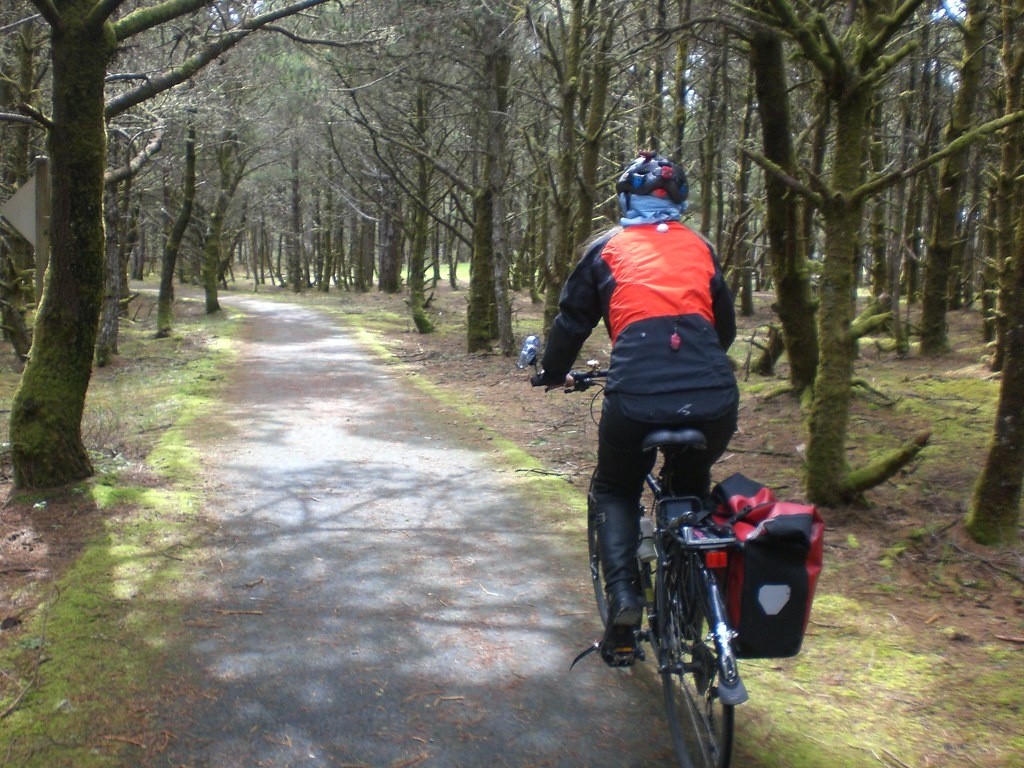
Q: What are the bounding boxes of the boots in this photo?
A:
[591,502,642,668]
[681,587,703,642]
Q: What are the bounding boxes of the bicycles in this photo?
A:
[515,333,749,767]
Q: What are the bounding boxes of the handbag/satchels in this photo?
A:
[710,473,824,659]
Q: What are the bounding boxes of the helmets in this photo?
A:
[616,151,689,204]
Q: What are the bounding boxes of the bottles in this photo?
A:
[636,505,658,562]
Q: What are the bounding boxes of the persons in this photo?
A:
[543,153,739,668]
